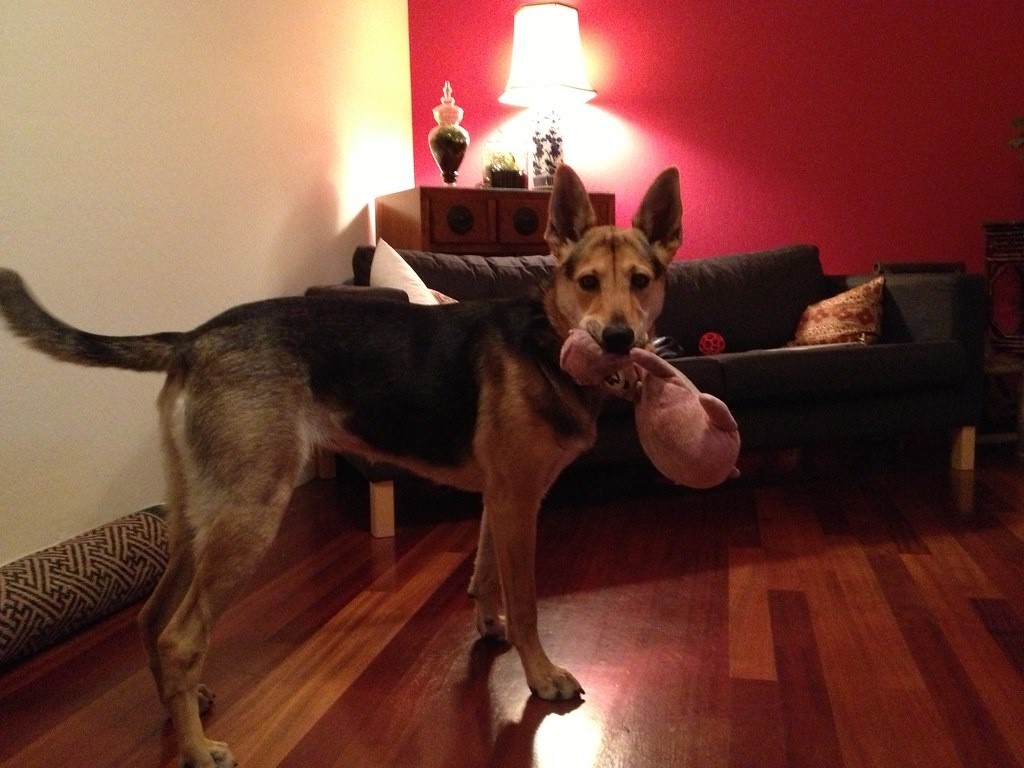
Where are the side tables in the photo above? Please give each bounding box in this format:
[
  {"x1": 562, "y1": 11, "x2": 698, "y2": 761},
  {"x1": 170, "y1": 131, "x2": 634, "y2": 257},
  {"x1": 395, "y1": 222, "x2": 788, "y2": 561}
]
[{"x1": 374, "y1": 185, "x2": 616, "y2": 256}]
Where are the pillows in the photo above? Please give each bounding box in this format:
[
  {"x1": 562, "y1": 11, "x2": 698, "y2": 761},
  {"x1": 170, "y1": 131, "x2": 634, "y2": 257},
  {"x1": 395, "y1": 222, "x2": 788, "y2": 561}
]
[
  {"x1": 369, "y1": 237, "x2": 459, "y2": 305},
  {"x1": 781, "y1": 276, "x2": 885, "y2": 347}
]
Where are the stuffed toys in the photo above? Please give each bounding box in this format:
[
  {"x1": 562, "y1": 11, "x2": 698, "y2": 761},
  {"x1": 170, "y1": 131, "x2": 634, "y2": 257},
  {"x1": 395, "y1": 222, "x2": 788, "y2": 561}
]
[{"x1": 558, "y1": 328, "x2": 741, "y2": 490}]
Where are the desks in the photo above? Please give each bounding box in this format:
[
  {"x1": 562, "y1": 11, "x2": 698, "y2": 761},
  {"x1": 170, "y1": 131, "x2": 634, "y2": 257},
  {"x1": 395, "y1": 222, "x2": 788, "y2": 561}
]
[{"x1": 984, "y1": 222, "x2": 1024, "y2": 409}]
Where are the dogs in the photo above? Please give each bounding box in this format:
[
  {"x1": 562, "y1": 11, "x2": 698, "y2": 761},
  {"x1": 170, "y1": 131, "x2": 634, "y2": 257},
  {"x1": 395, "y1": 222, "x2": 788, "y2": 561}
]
[{"x1": 2, "y1": 161, "x2": 681, "y2": 768}]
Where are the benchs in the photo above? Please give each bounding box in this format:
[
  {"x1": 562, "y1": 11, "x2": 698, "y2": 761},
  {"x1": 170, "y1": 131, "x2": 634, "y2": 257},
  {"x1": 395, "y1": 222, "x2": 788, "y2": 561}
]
[{"x1": 303, "y1": 242, "x2": 988, "y2": 539}]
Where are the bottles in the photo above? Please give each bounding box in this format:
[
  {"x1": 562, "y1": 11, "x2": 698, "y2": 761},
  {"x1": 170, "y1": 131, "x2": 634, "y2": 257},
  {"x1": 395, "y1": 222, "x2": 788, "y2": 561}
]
[{"x1": 427, "y1": 81, "x2": 471, "y2": 188}]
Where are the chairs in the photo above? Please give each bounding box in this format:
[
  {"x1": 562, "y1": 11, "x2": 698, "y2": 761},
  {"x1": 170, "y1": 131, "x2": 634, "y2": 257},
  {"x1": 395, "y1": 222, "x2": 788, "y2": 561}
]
[{"x1": 872, "y1": 259, "x2": 1024, "y2": 457}]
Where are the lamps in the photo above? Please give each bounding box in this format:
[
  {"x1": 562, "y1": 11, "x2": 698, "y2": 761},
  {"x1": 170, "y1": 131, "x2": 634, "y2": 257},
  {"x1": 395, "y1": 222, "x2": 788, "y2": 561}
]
[{"x1": 498, "y1": 1, "x2": 599, "y2": 190}]
